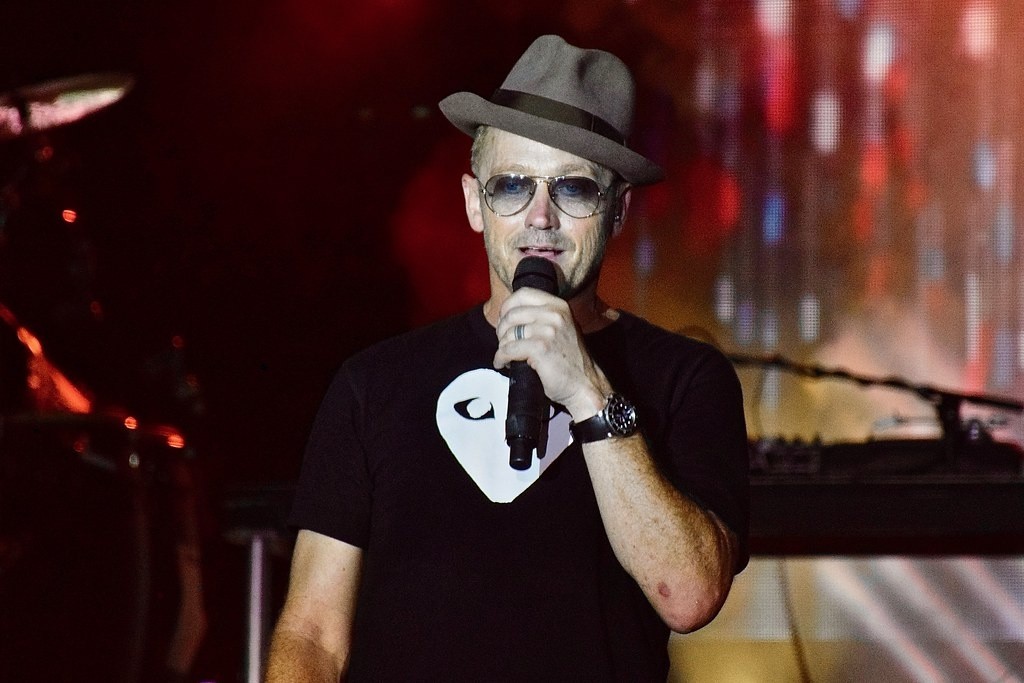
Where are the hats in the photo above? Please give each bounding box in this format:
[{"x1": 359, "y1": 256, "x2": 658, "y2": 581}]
[{"x1": 438, "y1": 35, "x2": 662, "y2": 186}]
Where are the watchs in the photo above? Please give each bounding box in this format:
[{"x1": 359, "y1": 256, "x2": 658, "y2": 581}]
[{"x1": 568, "y1": 391, "x2": 652, "y2": 444}]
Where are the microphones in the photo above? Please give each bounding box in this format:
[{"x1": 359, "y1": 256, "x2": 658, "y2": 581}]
[{"x1": 505, "y1": 255, "x2": 560, "y2": 471}]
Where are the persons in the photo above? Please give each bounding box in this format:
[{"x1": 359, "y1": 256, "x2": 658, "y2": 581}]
[{"x1": 261, "y1": 34, "x2": 751, "y2": 683}]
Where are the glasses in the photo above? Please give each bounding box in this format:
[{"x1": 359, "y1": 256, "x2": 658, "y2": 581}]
[{"x1": 477, "y1": 173, "x2": 614, "y2": 219}]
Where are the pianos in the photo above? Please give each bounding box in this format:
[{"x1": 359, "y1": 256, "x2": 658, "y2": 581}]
[{"x1": 735, "y1": 427, "x2": 1024, "y2": 561}]
